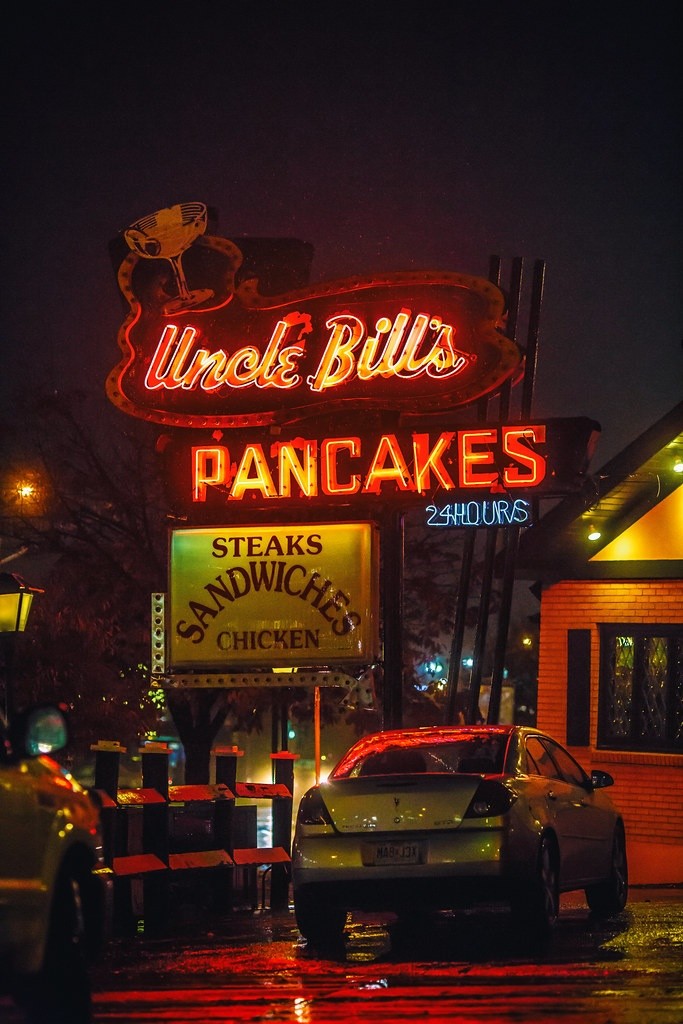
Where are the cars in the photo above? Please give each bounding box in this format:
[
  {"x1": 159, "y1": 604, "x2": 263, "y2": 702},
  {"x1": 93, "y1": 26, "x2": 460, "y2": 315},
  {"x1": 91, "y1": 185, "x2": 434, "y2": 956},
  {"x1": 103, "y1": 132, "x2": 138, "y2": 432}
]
[
  {"x1": 289, "y1": 723, "x2": 630, "y2": 941},
  {"x1": 0, "y1": 679, "x2": 121, "y2": 1024}
]
